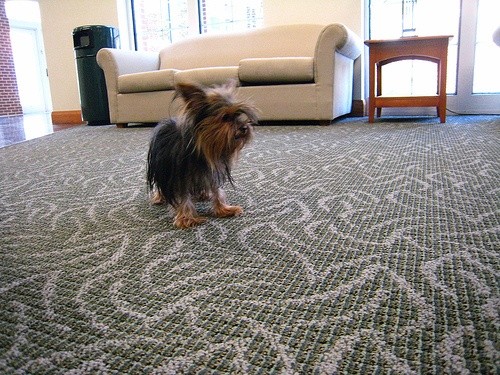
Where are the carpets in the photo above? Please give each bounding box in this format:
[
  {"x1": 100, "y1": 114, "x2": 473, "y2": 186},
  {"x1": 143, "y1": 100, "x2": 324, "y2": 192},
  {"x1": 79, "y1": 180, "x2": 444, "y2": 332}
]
[{"x1": 1, "y1": 116, "x2": 499, "y2": 375}]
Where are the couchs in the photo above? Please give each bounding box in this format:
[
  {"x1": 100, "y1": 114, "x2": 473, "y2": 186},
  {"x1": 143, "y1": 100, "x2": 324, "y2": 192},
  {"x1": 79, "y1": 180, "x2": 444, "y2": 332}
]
[{"x1": 96, "y1": 20, "x2": 362, "y2": 129}]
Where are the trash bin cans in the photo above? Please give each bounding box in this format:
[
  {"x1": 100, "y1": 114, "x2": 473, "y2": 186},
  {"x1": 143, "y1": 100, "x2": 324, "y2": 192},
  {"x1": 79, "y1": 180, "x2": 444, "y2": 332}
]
[{"x1": 72, "y1": 24, "x2": 120, "y2": 125}]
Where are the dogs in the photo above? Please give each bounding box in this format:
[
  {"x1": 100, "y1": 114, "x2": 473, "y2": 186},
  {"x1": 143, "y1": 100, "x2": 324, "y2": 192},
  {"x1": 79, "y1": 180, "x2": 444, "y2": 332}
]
[{"x1": 145, "y1": 77, "x2": 264, "y2": 231}]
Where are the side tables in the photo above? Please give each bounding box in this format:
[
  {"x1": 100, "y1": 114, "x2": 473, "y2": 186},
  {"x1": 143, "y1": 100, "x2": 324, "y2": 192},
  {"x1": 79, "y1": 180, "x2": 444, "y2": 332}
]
[{"x1": 364, "y1": 33, "x2": 455, "y2": 125}]
